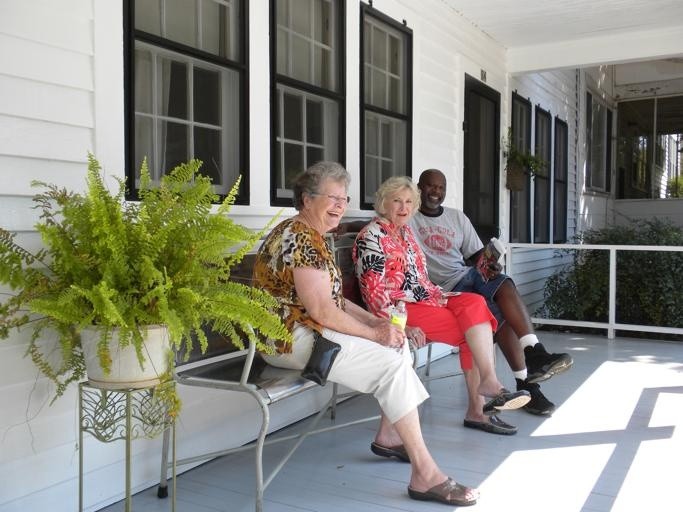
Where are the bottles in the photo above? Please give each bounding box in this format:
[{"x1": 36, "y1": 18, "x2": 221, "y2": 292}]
[{"x1": 389, "y1": 302, "x2": 407, "y2": 331}]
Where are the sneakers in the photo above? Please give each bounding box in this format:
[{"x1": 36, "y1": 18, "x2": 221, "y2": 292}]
[
  {"x1": 482, "y1": 389, "x2": 531, "y2": 415},
  {"x1": 464, "y1": 413, "x2": 518, "y2": 434}
]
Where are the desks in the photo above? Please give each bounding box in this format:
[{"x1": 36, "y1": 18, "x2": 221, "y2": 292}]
[{"x1": 77, "y1": 384, "x2": 178, "y2": 512}]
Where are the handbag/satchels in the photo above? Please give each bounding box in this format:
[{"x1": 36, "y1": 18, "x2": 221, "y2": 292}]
[{"x1": 301, "y1": 330, "x2": 341, "y2": 387}]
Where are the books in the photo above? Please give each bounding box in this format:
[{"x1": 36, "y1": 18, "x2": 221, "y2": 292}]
[{"x1": 475, "y1": 237, "x2": 506, "y2": 285}]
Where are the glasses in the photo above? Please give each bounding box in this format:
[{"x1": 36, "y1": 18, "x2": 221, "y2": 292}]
[{"x1": 310, "y1": 191, "x2": 348, "y2": 205}]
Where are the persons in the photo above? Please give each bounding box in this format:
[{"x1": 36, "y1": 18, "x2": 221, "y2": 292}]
[
  {"x1": 247, "y1": 159, "x2": 480, "y2": 505},
  {"x1": 353, "y1": 174, "x2": 531, "y2": 436},
  {"x1": 407, "y1": 169, "x2": 575, "y2": 416}
]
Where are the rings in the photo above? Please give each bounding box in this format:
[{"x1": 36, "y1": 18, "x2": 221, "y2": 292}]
[
  {"x1": 492, "y1": 273, "x2": 494, "y2": 277},
  {"x1": 414, "y1": 333, "x2": 417, "y2": 337}
]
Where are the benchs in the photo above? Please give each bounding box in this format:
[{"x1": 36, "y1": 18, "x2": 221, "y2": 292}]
[{"x1": 158, "y1": 241, "x2": 495, "y2": 511}]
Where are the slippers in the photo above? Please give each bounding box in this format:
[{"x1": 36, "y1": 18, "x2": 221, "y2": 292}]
[
  {"x1": 371, "y1": 442, "x2": 412, "y2": 463},
  {"x1": 407, "y1": 477, "x2": 480, "y2": 505}
]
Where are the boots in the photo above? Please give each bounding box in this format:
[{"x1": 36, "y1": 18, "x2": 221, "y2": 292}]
[
  {"x1": 514, "y1": 376, "x2": 555, "y2": 416},
  {"x1": 523, "y1": 343, "x2": 572, "y2": 384}
]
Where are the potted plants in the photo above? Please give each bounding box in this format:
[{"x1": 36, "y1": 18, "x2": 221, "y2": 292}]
[{"x1": 1, "y1": 149, "x2": 297, "y2": 455}]
